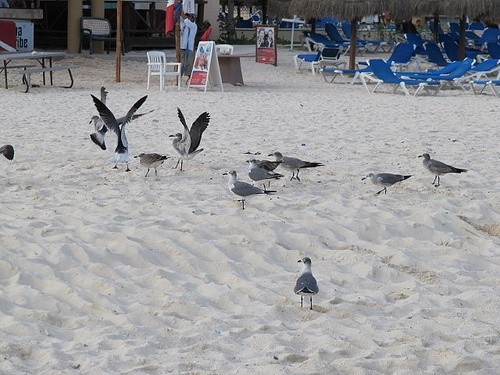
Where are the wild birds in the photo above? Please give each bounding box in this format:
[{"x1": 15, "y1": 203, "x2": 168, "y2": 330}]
[
  {"x1": 361, "y1": 173, "x2": 413, "y2": 194},
  {"x1": 88, "y1": 86, "x2": 207, "y2": 176},
  {"x1": 221, "y1": 152, "x2": 326, "y2": 209},
  {"x1": 418, "y1": 153, "x2": 470, "y2": 189},
  {"x1": 293, "y1": 256, "x2": 320, "y2": 311},
  {"x1": 0, "y1": 144, "x2": 14, "y2": 162}
]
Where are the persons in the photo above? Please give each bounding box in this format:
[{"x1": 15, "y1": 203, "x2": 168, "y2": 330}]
[
  {"x1": 180, "y1": 10, "x2": 198, "y2": 76},
  {"x1": 257, "y1": 29, "x2": 273, "y2": 47},
  {"x1": 415, "y1": 20, "x2": 422, "y2": 28}
]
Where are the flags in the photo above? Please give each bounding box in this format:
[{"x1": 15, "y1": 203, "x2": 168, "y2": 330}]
[{"x1": 165, "y1": 0, "x2": 174, "y2": 35}]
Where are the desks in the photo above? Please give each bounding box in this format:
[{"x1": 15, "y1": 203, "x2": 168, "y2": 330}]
[
  {"x1": 218, "y1": 53, "x2": 244, "y2": 86},
  {"x1": 0, "y1": 51, "x2": 66, "y2": 92}
]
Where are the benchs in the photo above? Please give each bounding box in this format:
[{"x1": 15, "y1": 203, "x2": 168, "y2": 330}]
[
  {"x1": 78, "y1": 17, "x2": 125, "y2": 56},
  {"x1": 1, "y1": 62, "x2": 81, "y2": 93}
]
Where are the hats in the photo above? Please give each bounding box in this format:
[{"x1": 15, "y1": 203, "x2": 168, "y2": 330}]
[{"x1": 186, "y1": 13, "x2": 196, "y2": 18}]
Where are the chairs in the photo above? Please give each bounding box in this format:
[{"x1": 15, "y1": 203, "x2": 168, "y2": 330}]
[
  {"x1": 147, "y1": 50, "x2": 183, "y2": 92},
  {"x1": 237, "y1": 18, "x2": 500, "y2": 96}
]
